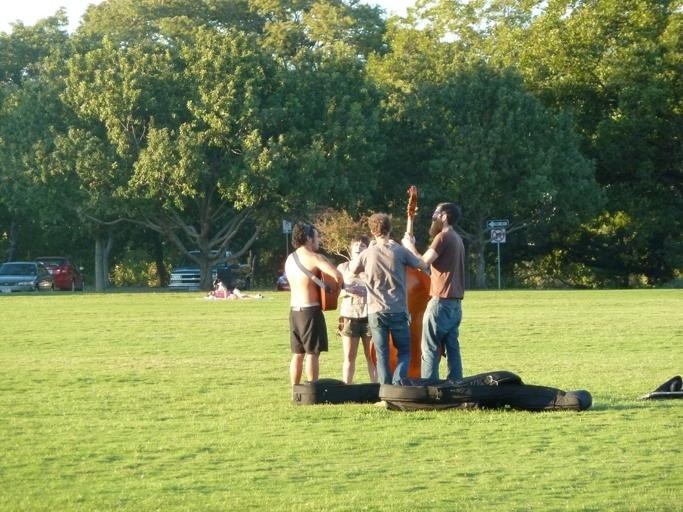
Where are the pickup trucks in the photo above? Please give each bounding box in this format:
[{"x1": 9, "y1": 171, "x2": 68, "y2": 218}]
[{"x1": 169, "y1": 248, "x2": 253, "y2": 294}]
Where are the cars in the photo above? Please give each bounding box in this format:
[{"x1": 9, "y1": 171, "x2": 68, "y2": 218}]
[
  {"x1": 277, "y1": 274, "x2": 293, "y2": 292},
  {"x1": 0, "y1": 261, "x2": 57, "y2": 294},
  {"x1": 34, "y1": 256, "x2": 87, "y2": 293}
]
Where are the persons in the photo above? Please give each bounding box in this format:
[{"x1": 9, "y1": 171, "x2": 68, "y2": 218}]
[
  {"x1": 398, "y1": 201, "x2": 467, "y2": 382},
  {"x1": 282, "y1": 221, "x2": 345, "y2": 401},
  {"x1": 206, "y1": 287, "x2": 263, "y2": 300},
  {"x1": 333, "y1": 236, "x2": 378, "y2": 384},
  {"x1": 349, "y1": 213, "x2": 425, "y2": 384}
]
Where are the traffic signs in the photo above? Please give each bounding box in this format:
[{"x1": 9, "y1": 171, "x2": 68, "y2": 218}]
[{"x1": 487, "y1": 219, "x2": 510, "y2": 230}]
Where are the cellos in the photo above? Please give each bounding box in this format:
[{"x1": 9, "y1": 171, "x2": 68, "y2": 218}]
[{"x1": 369, "y1": 186, "x2": 442, "y2": 378}]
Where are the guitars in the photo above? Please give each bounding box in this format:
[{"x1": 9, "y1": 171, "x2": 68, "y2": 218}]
[{"x1": 319, "y1": 271, "x2": 337, "y2": 310}]
